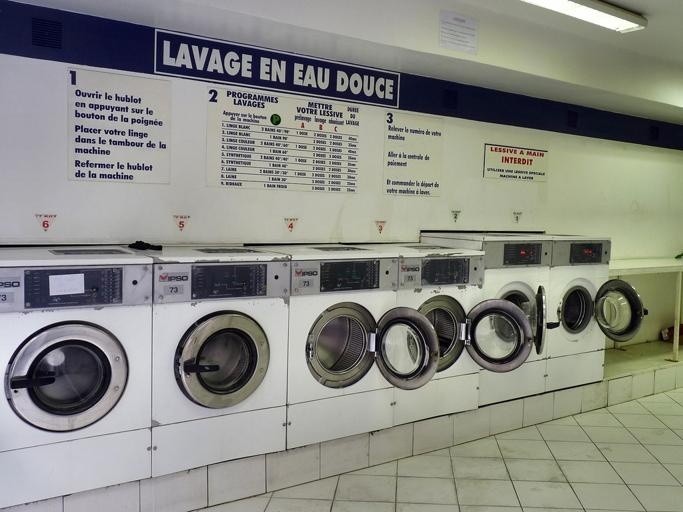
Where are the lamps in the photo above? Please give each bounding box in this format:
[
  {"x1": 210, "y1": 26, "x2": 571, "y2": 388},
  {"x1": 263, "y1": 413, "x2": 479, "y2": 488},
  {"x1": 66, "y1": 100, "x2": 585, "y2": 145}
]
[{"x1": 520, "y1": 1, "x2": 648, "y2": 36}]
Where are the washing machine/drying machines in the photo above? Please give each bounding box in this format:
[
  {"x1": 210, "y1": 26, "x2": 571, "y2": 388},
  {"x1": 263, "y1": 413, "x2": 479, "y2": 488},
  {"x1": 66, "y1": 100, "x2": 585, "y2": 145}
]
[
  {"x1": 242, "y1": 245, "x2": 441, "y2": 451},
  {"x1": 527, "y1": 233, "x2": 648, "y2": 395},
  {"x1": 1, "y1": 246, "x2": 154, "y2": 510},
  {"x1": 127, "y1": 245, "x2": 291, "y2": 480},
  {"x1": 350, "y1": 243, "x2": 534, "y2": 426},
  {"x1": 421, "y1": 232, "x2": 562, "y2": 406}
]
